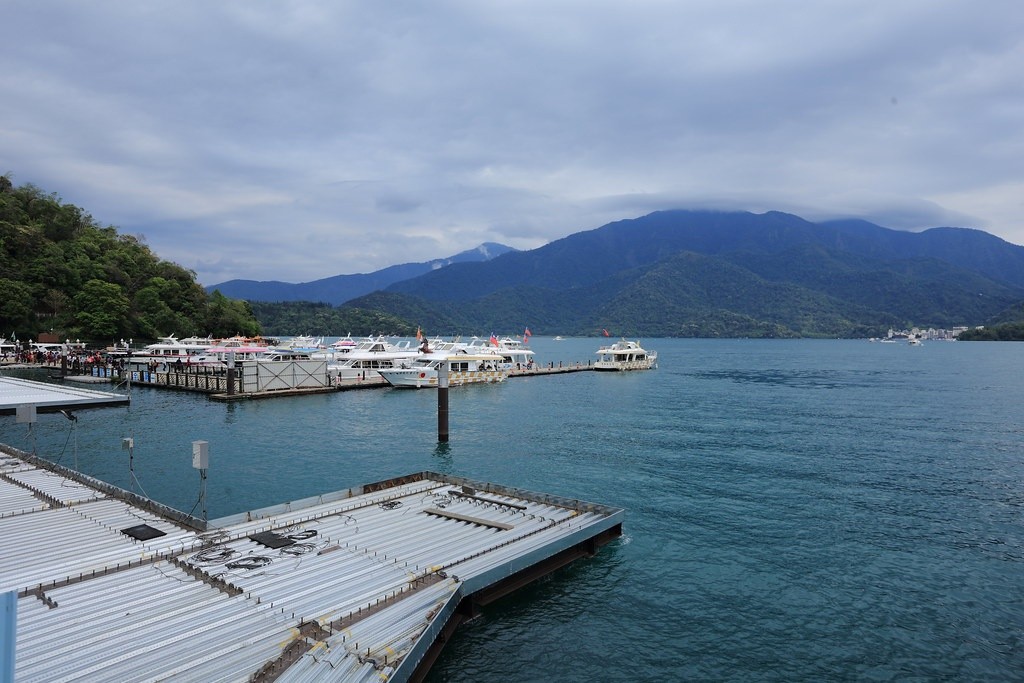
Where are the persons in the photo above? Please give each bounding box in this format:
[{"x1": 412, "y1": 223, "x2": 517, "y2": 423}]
[
  {"x1": 479, "y1": 364, "x2": 497, "y2": 371},
  {"x1": 187, "y1": 349, "x2": 195, "y2": 355},
  {"x1": 149, "y1": 358, "x2": 158, "y2": 374},
  {"x1": 163, "y1": 357, "x2": 167, "y2": 372},
  {"x1": 527, "y1": 357, "x2": 533, "y2": 369},
  {"x1": 186, "y1": 357, "x2": 191, "y2": 370},
  {"x1": 176, "y1": 357, "x2": 181, "y2": 371},
  {"x1": 15, "y1": 350, "x2": 126, "y2": 368},
  {"x1": 2, "y1": 351, "x2": 8, "y2": 362}
]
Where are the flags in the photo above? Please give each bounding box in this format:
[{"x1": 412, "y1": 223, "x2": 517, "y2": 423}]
[
  {"x1": 11, "y1": 332, "x2": 15, "y2": 341},
  {"x1": 524, "y1": 327, "x2": 531, "y2": 343},
  {"x1": 602, "y1": 329, "x2": 609, "y2": 337},
  {"x1": 490, "y1": 332, "x2": 499, "y2": 347}
]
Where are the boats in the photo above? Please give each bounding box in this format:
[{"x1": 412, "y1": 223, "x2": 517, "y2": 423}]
[
  {"x1": 144, "y1": 333, "x2": 534, "y2": 376},
  {"x1": 593, "y1": 336, "x2": 658, "y2": 371},
  {"x1": 376, "y1": 352, "x2": 510, "y2": 390},
  {"x1": 326, "y1": 341, "x2": 425, "y2": 386},
  {"x1": 879, "y1": 337, "x2": 896, "y2": 344},
  {"x1": 907, "y1": 340, "x2": 924, "y2": 347},
  {"x1": 869, "y1": 338, "x2": 875, "y2": 342}
]
